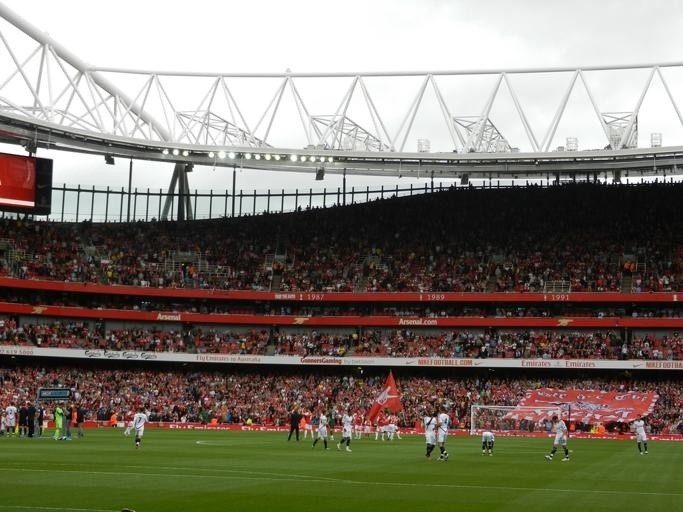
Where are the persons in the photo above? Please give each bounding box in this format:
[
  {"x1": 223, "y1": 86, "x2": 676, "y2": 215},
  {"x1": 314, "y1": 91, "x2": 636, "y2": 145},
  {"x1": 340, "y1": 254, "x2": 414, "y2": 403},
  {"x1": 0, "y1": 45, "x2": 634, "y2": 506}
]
[
  {"x1": 431, "y1": 404, "x2": 449, "y2": 463},
  {"x1": 630, "y1": 414, "x2": 649, "y2": 455},
  {"x1": 0, "y1": 293, "x2": 683, "y2": 441},
  {"x1": 335, "y1": 409, "x2": 352, "y2": 453},
  {"x1": 541, "y1": 414, "x2": 569, "y2": 461},
  {"x1": 421, "y1": 408, "x2": 436, "y2": 460},
  {"x1": 310, "y1": 409, "x2": 331, "y2": 450},
  {"x1": 132, "y1": 405, "x2": 148, "y2": 449},
  {"x1": 480, "y1": 430, "x2": 494, "y2": 456},
  {"x1": 1, "y1": 179, "x2": 682, "y2": 293}
]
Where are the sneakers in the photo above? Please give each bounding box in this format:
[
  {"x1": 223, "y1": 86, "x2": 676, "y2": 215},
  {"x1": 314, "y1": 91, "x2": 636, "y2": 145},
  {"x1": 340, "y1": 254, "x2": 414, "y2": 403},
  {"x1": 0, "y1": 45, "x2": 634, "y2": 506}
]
[
  {"x1": 424, "y1": 450, "x2": 448, "y2": 462},
  {"x1": 336, "y1": 444, "x2": 341, "y2": 451},
  {"x1": 0, "y1": 430, "x2": 83, "y2": 441},
  {"x1": 560, "y1": 458, "x2": 568, "y2": 462},
  {"x1": 354, "y1": 436, "x2": 402, "y2": 441},
  {"x1": 544, "y1": 456, "x2": 552, "y2": 461},
  {"x1": 346, "y1": 447, "x2": 352, "y2": 452}
]
